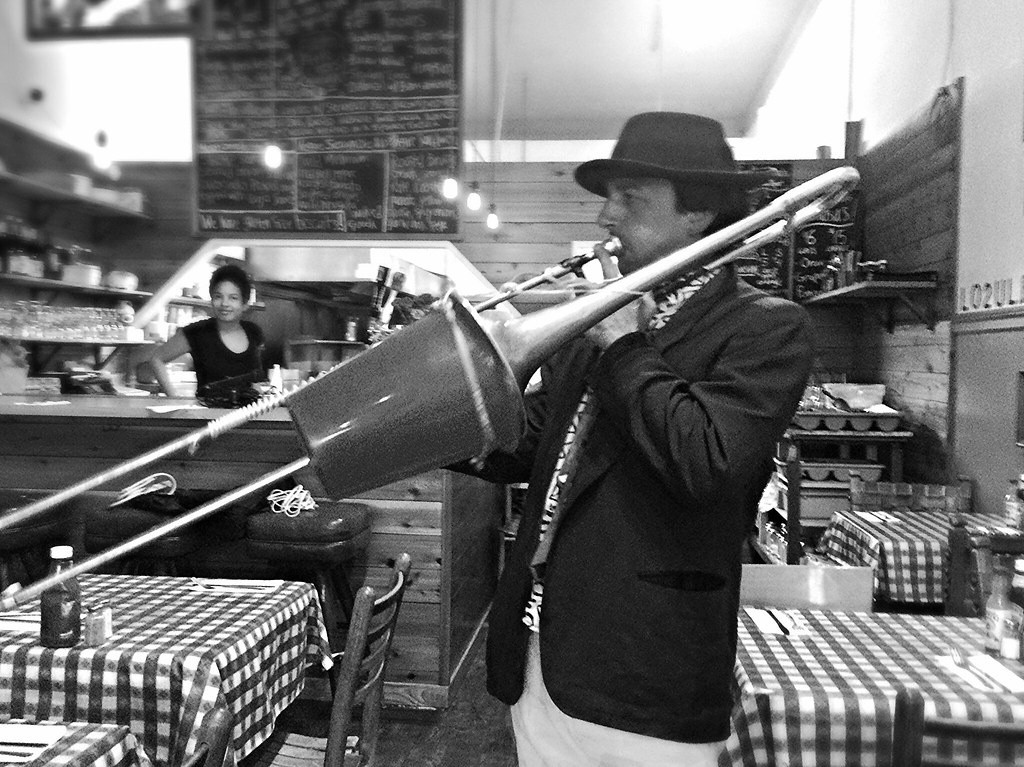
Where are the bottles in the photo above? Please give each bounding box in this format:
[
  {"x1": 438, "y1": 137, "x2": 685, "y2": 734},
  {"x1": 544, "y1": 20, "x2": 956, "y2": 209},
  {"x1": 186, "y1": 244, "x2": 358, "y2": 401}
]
[
  {"x1": 41, "y1": 546, "x2": 81, "y2": 649},
  {"x1": 986, "y1": 582, "x2": 1011, "y2": 652},
  {"x1": 1003, "y1": 480, "x2": 1024, "y2": 529},
  {"x1": 0, "y1": 216, "x2": 44, "y2": 278}
]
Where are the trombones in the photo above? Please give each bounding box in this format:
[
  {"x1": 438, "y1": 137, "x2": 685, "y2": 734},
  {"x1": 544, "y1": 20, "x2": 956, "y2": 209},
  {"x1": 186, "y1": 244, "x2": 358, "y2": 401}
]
[{"x1": 1, "y1": 164, "x2": 861, "y2": 618}]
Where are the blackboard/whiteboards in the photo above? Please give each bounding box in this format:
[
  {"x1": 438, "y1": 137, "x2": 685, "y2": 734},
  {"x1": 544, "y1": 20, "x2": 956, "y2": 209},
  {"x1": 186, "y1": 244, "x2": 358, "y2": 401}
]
[
  {"x1": 189, "y1": 2, "x2": 467, "y2": 241},
  {"x1": 737, "y1": 164, "x2": 795, "y2": 301},
  {"x1": 797, "y1": 195, "x2": 866, "y2": 299}
]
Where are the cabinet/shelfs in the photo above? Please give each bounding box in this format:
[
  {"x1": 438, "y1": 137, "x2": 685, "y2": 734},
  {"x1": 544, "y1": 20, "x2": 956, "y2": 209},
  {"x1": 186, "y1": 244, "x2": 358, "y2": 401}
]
[
  {"x1": 0, "y1": 168, "x2": 155, "y2": 297},
  {"x1": 747, "y1": 430, "x2": 920, "y2": 564}
]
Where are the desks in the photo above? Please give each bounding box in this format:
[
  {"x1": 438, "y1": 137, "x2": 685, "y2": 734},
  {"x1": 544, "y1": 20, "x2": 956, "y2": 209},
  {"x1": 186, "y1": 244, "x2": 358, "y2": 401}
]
[
  {"x1": 720, "y1": 606, "x2": 1024, "y2": 767},
  {"x1": 0, "y1": 335, "x2": 156, "y2": 403},
  {"x1": 817, "y1": 511, "x2": 1024, "y2": 605},
  {"x1": 0, "y1": 571, "x2": 334, "y2": 767}
]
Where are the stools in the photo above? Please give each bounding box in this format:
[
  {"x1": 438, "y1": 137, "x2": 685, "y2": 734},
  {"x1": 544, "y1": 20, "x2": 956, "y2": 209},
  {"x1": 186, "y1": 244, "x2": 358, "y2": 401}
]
[
  {"x1": 246, "y1": 502, "x2": 371, "y2": 703},
  {"x1": 81, "y1": 511, "x2": 165, "y2": 576},
  {"x1": 0, "y1": 490, "x2": 66, "y2": 590}
]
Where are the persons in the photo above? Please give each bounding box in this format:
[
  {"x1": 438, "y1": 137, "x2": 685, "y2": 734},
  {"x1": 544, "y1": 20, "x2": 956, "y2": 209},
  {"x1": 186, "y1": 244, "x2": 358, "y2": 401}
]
[
  {"x1": 442, "y1": 112, "x2": 817, "y2": 767},
  {"x1": 149, "y1": 264, "x2": 266, "y2": 412}
]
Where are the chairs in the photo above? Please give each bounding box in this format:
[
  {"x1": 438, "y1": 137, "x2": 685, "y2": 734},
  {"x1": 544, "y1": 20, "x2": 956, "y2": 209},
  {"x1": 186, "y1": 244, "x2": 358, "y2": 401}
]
[
  {"x1": 894, "y1": 691, "x2": 1024, "y2": 767},
  {"x1": 848, "y1": 470, "x2": 972, "y2": 512},
  {"x1": 740, "y1": 564, "x2": 876, "y2": 615},
  {"x1": 237, "y1": 554, "x2": 412, "y2": 766},
  {"x1": 944, "y1": 518, "x2": 1024, "y2": 615}
]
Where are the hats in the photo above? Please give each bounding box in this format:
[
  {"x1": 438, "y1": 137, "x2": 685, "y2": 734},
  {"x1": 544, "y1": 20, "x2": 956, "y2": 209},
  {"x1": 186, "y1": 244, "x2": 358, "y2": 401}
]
[{"x1": 574, "y1": 111, "x2": 778, "y2": 199}]
